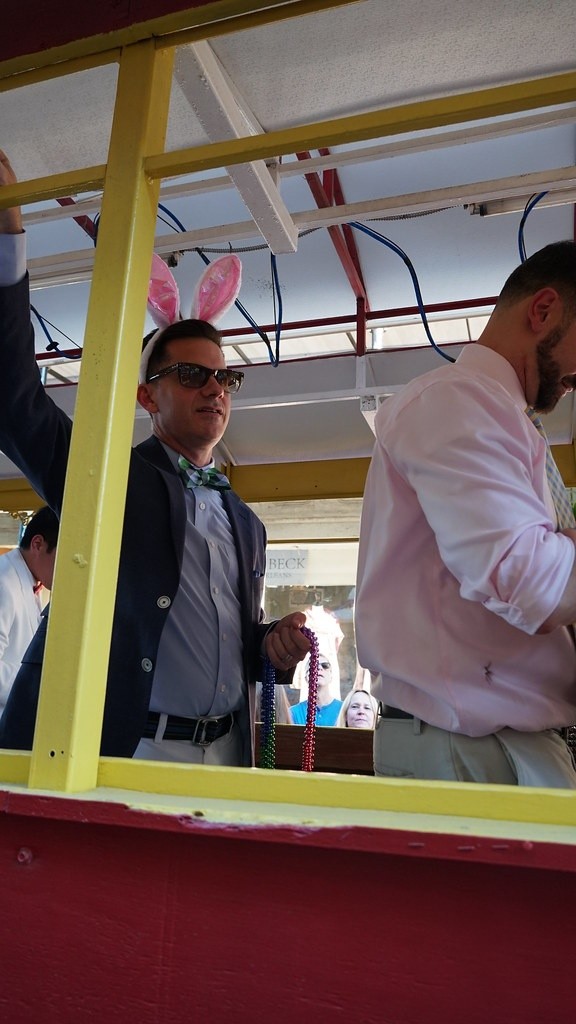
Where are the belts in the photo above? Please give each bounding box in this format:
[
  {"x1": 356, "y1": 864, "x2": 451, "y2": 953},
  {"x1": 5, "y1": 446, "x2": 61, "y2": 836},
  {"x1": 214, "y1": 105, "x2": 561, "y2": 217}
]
[
  {"x1": 141, "y1": 711, "x2": 239, "y2": 745},
  {"x1": 379, "y1": 701, "x2": 414, "y2": 718}
]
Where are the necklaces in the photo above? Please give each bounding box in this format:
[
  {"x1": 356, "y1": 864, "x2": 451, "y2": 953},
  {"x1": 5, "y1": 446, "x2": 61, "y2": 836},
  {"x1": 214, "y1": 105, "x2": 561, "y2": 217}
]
[{"x1": 258, "y1": 625, "x2": 320, "y2": 773}]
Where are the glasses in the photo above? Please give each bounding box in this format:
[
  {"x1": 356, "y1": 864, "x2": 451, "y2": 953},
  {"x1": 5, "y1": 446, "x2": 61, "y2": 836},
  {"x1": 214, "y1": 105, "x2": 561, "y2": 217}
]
[
  {"x1": 306, "y1": 662, "x2": 331, "y2": 672},
  {"x1": 147, "y1": 363, "x2": 245, "y2": 394}
]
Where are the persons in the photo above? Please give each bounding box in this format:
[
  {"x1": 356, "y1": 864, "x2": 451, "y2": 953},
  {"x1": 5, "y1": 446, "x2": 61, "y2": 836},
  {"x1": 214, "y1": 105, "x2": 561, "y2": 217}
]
[
  {"x1": 353, "y1": 237, "x2": 576, "y2": 794},
  {"x1": 0, "y1": 505, "x2": 60, "y2": 716},
  {"x1": 1, "y1": 149, "x2": 315, "y2": 769},
  {"x1": 288, "y1": 653, "x2": 349, "y2": 729},
  {"x1": 333, "y1": 687, "x2": 381, "y2": 731}
]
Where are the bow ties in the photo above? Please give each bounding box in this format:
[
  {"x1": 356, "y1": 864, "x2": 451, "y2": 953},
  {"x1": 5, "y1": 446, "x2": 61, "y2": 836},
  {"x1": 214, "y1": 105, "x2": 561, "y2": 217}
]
[{"x1": 178, "y1": 454, "x2": 231, "y2": 490}]
